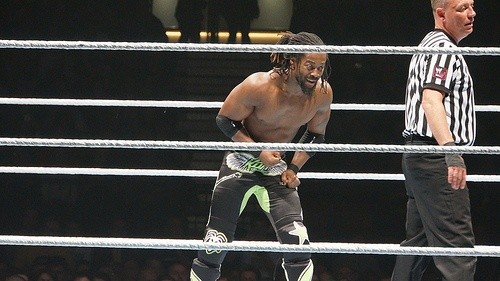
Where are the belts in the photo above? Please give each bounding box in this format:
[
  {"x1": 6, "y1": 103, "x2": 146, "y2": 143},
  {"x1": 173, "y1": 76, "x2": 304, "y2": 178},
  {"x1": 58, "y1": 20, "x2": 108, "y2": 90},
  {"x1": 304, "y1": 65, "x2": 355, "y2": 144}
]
[{"x1": 404, "y1": 136, "x2": 436, "y2": 143}]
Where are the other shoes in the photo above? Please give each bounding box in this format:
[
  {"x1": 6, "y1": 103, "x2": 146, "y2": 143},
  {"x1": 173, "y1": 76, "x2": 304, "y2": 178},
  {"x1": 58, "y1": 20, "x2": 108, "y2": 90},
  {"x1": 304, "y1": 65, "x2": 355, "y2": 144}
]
[
  {"x1": 179, "y1": 34, "x2": 200, "y2": 43},
  {"x1": 241, "y1": 34, "x2": 251, "y2": 44},
  {"x1": 226, "y1": 34, "x2": 236, "y2": 44}
]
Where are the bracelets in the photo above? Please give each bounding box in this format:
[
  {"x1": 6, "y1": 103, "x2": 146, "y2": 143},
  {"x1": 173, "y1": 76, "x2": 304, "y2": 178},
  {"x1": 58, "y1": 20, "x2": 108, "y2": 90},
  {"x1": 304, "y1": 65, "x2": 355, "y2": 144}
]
[
  {"x1": 250, "y1": 150, "x2": 262, "y2": 158},
  {"x1": 288, "y1": 164, "x2": 299, "y2": 174}
]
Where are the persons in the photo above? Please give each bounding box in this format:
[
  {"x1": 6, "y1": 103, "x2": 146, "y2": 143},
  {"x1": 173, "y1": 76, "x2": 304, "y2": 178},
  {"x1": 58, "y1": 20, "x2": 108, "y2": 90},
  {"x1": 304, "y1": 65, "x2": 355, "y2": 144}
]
[
  {"x1": 190, "y1": 31, "x2": 334, "y2": 281},
  {"x1": 0, "y1": 212, "x2": 391, "y2": 281},
  {"x1": 174, "y1": 0, "x2": 260, "y2": 43},
  {"x1": 390, "y1": 0, "x2": 477, "y2": 281}
]
